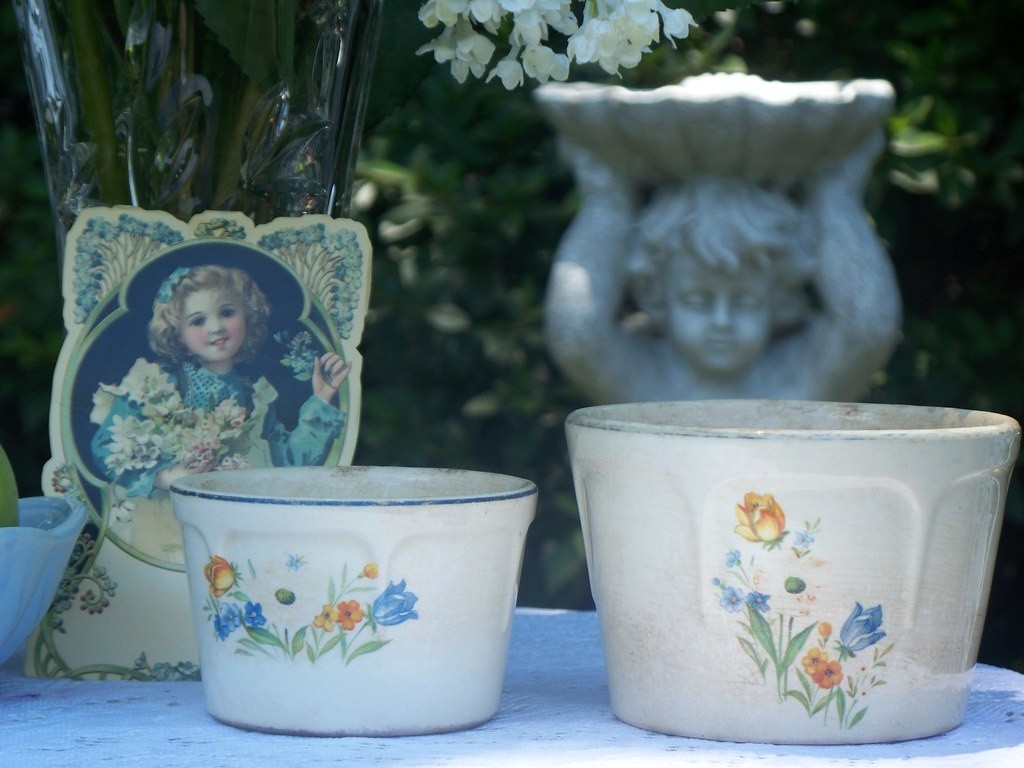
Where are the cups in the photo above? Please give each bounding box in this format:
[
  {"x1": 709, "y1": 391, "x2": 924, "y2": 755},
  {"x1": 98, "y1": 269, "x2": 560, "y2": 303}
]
[{"x1": 9, "y1": 0, "x2": 390, "y2": 258}]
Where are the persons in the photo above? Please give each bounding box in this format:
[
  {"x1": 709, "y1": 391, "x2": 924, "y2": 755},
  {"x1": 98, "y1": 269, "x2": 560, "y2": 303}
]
[{"x1": 544, "y1": 133, "x2": 900, "y2": 402}]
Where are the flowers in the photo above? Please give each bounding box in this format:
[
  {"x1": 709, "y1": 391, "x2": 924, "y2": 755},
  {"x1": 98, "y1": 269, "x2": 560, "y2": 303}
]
[{"x1": 415, "y1": 0, "x2": 698, "y2": 91}]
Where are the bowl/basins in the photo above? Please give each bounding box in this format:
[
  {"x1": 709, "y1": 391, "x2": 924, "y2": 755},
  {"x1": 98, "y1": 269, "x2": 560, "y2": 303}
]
[
  {"x1": 0, "y1": 495, "x2": 90, "y2": 663},
  {"x1": 559, "y1": 397, "x2": 1022, "y2": 750},
  {"x1": 164, "y1": 462, "x2": 544, "y2": 740}
]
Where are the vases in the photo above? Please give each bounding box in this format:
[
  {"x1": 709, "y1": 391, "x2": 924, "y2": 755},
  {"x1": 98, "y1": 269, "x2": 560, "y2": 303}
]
[{"x1": 12, "y1": 0, "x2": 382, "y2": 272}]
